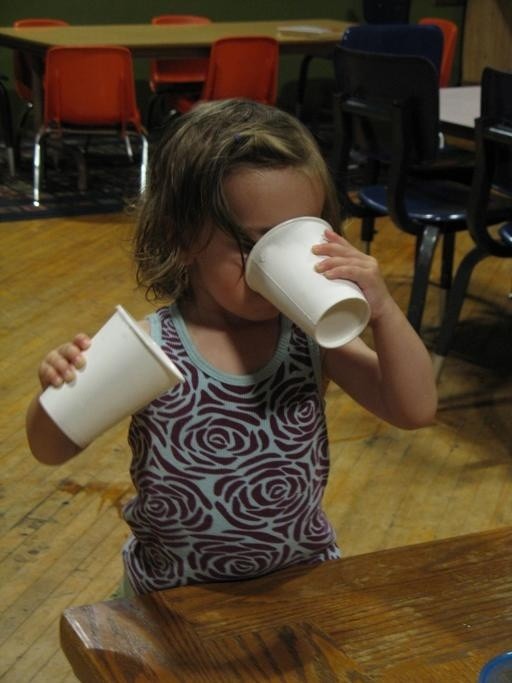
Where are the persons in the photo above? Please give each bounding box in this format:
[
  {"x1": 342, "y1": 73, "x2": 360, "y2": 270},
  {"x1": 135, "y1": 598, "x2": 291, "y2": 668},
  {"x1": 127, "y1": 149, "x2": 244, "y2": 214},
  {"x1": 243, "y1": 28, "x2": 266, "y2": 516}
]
[{"x1": 23, "y1": 93, "x2": 442, "y2": 599}]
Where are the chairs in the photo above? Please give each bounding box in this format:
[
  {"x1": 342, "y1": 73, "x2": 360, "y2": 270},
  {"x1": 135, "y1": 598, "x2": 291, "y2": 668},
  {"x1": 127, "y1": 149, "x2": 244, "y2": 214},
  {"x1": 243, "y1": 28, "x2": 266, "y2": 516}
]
[
  {"x1": 146, "y1": 15, "x2": 215, "y2": 123},
  {"x1": 435, "y1": 66, "x2": 509, "y2": 354},
  {"x1": 28, "y1": 45, "x2": 154, "y2": 211},
  {"x1": 166, "y1": 35, "x2": 281, "y2": 123},
  {"x1": 296, "y1": 18, "x2": 459, "y2": 118},
  {"x1": 10, "y1": 19, "x2": 93, "y2": 195},
  {"x1": 327, "y1": 23, "x2": 479, "y2": 334}
]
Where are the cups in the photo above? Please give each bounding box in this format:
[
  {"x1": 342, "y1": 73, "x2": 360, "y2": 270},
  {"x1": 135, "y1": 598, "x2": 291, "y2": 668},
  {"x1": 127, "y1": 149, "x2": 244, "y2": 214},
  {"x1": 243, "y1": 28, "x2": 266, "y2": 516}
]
[
  {"x1": 38, "y1": 303, "x2": 188, "y2": 449},
  {"x1": 243, "y1": 217, "x2": 373, "y2": 350}
]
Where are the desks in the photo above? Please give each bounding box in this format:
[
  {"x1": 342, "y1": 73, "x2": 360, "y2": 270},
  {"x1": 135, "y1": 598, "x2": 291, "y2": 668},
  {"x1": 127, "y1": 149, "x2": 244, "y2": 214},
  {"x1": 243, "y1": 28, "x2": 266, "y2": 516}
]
[
  {"x1": 2, "y1": 13, "x2": 349, "y2": 192},
  {"x1": 432, "y1": 82, "x2": 511, "y2": 291},
  {"x1": 57, "y1": 526, "x2": 511, "y2": 682}
]
